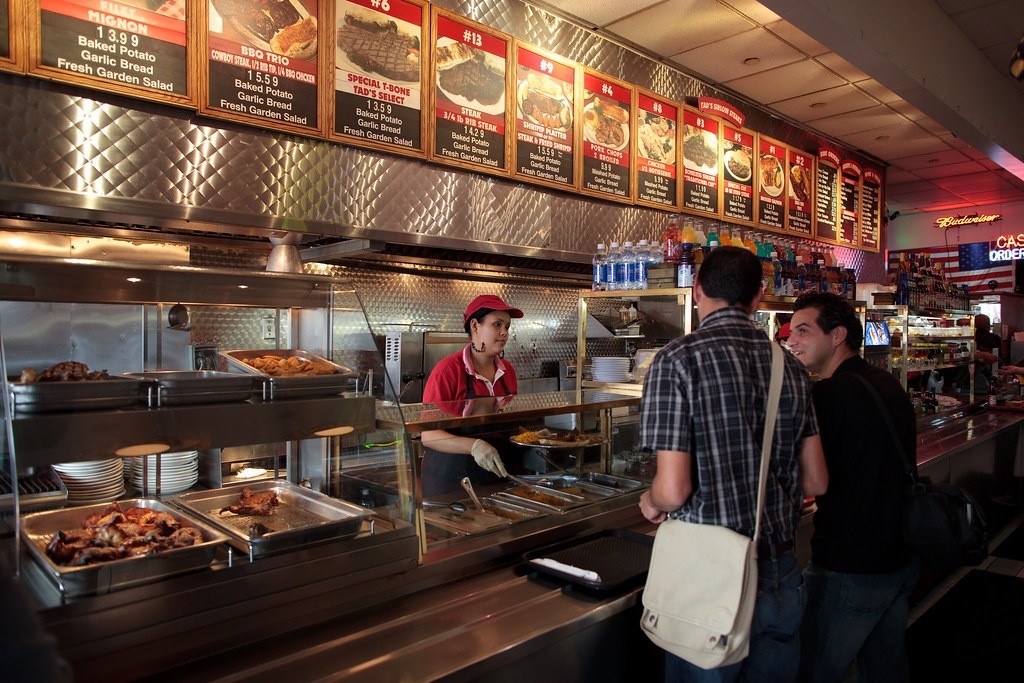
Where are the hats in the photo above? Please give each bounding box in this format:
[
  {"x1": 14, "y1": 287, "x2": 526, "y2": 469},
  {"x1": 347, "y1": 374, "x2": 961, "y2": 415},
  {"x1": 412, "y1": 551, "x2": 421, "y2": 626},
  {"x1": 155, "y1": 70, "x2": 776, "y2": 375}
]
[
  {"x1": 464, "y1": 295, "x2": 524, "y2": 326},
  {"x1": 775, "y1": 323, "x2": 791, "y2": 339}
]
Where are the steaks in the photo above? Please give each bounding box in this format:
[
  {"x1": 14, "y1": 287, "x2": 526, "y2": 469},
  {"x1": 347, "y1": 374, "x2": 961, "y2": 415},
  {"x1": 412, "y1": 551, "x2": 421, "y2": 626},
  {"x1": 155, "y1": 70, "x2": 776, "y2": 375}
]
[
  {"x1": 683, "y1": 140, "x2": 717, "y2": 168},
  {"x1": 527, "y1": 91, "x2": 560, "y2": 115},
  {"x1": 728, "y1": 160, "x2": 750, "y2": 178},
  {"x1": 336, "y1": 25, "x2": 420, "y2": 82},
  {"x1": 440, "y1": 61, "x2": 505, "y2": 105}
]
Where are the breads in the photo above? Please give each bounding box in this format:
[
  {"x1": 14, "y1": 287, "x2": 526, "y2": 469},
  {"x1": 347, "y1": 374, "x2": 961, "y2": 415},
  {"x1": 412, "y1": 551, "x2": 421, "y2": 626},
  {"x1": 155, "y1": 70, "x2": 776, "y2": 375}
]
[
  {"x1": 519, "y1": 426, "x2": 557, "y2": 438},
  {"x1": 539, "y1": 438, "x2": 589, "y2": 445},
  {"x1": 592, "y1": 98, "x2": 629, "y2": 124},
  {"x1": 271, "y1": 15, "x2": 318, "y2": 60}
]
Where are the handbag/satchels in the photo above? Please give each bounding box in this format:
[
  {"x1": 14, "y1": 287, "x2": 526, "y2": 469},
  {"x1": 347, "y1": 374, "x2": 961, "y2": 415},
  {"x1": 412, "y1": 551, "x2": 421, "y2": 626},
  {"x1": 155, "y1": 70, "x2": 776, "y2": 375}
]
[{"x1": 640, "y1": 519, "x2": 758, "y2": 670}]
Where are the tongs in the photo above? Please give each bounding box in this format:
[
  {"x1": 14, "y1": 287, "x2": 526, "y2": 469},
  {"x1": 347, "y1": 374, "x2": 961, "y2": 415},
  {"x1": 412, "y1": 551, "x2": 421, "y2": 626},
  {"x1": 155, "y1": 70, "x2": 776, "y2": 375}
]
[
  {"x1": 422, "y1": 500, "x2": 466, "y2": 512},
  {"x1": 517, "y1": 472, "x2": 580, "y2": 489}
]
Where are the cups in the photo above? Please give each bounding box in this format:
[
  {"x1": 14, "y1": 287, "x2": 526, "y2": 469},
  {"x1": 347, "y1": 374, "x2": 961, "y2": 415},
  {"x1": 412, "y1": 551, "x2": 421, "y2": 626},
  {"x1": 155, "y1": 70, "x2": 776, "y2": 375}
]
[
  {"x1": 628, "y1": 325, "x2": 639, "y2": 336},
  {"x1": 1014, "y1": 332, "x2": 1024, "y2": 341}
]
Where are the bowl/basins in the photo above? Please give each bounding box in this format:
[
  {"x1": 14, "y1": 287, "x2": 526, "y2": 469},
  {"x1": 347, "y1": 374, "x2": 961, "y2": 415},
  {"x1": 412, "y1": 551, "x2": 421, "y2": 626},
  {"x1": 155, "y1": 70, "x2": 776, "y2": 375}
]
[{"x1": 615, "y1": 329, "x2": 628, "y2": 335}]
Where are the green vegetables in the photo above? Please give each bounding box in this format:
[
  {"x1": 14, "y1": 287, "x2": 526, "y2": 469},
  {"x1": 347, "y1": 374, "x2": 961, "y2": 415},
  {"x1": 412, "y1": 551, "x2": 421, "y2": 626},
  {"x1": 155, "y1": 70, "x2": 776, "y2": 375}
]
[
  {"x1": 592, "y1": 106, "x2": 604, "y2": 116},
  {"x1": 644, "y1": 113, "x2": 672, "y2": 161},
  {"x1": 346, "y1": 15, "x2": 420, "y2": 72}
]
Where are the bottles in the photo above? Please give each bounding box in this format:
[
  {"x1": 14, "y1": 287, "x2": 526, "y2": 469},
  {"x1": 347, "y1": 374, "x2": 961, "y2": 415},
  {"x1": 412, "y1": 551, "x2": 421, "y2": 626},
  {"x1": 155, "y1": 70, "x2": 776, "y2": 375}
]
[
  {"x1": 988, "y1": 374, "x2": 1023, "y2": 405},
  {"x1": 592, "y1": 240, "x2": 663, "y2": 289},
  {"x1": 620, "y1": 303, "x2": 637, "y2": 329},
  {"x1": 663, "y1": 214, "x2": 856, "y2": 301},
  {"x1": 910, "y1": 387, "x2": 941, "y2": 418},
  {"x1": 896, "y1": 250, "x2": 970, "y2": 311}
]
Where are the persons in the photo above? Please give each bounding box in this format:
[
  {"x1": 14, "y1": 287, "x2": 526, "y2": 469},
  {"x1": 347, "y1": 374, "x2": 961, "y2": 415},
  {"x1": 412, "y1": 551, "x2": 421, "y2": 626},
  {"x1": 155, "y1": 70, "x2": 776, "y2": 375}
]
[
  {"x1": 786, "y1": 291, "x2": 920, "y2": 683},
  {"x1": 908, "y1": 309, "x2": 1024, "y2": 391},
  {"x1": 636, "y1": 242, "x2": 827, "y2": 683},
  {"x1": 420, "y1": 296, "x2": 526, "y2": 499}
]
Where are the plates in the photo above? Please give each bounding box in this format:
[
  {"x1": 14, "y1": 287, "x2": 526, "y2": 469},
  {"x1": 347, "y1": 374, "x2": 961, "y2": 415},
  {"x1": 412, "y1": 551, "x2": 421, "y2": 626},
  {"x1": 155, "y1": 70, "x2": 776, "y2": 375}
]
[
  {"x1": 724, "y1": 150, "x2": 751, "y2": 181},
  {"x1": 583, "y1": 102, "x2": 630, "y2": 151},
  {"x1": 211, "y1": 0, "x2": 422, "y2": 84},
  {"x1": 639, "y1": 123, "x2": 676, "y2": 164},
  {"x1": 53, "y1": 458, "x2": 127, "y2": 508},
  {"x1": 591, "y1": 391, "x2": 630, "y2": 403},
  {"x1": 683, "y1": 125, "x2": 718, "y2": 175},
  {"x1": 123, "y1": 450, "x2": 199, "y2": 496},
  {"x1": 760, "y1": 155, "x2": 785, "y2": 197},
  {"x1": 790, "y1": 165, "x2": 806, "y2": 199},
  {"x1": 591, "y1": 357, "x2": 634, "y2": 383},
  {"x1": 436, "y1": 36, "x2": 505, "y2": 115},
  {"x1": 517, "y1": 78, "x2": 573, "y2": 132}
]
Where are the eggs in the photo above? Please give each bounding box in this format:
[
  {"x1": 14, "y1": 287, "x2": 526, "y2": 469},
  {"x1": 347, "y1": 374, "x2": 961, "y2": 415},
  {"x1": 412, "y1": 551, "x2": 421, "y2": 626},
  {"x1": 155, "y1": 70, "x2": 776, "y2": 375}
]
[{"x1": 584, "y1": 108, "x2": 598, "y2": 127}]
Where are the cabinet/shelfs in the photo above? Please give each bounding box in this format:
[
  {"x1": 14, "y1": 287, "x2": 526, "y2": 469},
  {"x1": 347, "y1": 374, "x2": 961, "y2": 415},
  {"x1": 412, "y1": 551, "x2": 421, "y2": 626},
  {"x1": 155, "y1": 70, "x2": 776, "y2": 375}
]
[{"x1": 868, "y1": 304, "x2": 975, "y2": 404}]
